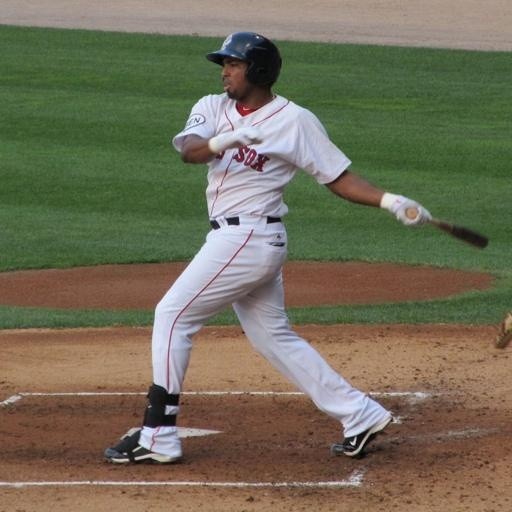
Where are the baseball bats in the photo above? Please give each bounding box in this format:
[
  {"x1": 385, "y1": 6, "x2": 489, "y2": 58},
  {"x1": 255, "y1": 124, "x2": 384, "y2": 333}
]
[{"x1": 405, "y1": 207, "x2": 489, "y2": 251}]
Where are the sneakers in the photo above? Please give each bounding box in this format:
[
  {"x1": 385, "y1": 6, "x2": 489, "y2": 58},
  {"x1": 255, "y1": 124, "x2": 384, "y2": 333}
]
[
  {"x1": 103, "y1": 427, "x2": 176, "y2": 464},
  {"x1": 342, "y1": 413, "x2": 393, "y2": 456}
]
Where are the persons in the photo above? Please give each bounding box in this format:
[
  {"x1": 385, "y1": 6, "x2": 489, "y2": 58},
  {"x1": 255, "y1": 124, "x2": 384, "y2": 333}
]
[{"x1": 102, "y1": 31, "x2": 434, "y2": 464}]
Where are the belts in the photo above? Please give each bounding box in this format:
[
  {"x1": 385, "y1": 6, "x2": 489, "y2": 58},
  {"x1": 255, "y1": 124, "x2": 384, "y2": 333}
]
[{"x1": 209, "y1": 215, "x2": 281, "y2": 230}]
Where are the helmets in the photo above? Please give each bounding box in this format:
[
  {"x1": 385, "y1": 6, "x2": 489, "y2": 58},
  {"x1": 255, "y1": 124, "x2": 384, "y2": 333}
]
[{"x1": 206, "y1": 31, "x2": 281, "y2": 87}]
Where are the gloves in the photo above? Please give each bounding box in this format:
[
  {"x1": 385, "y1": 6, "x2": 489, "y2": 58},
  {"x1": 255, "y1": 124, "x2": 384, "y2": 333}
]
[
  {"x1": 379, "y1": 191, "x2": 433, "y2": 226},
  {"x1": 208, "y1": 127, "x2": 264, "y2": 154}
]
